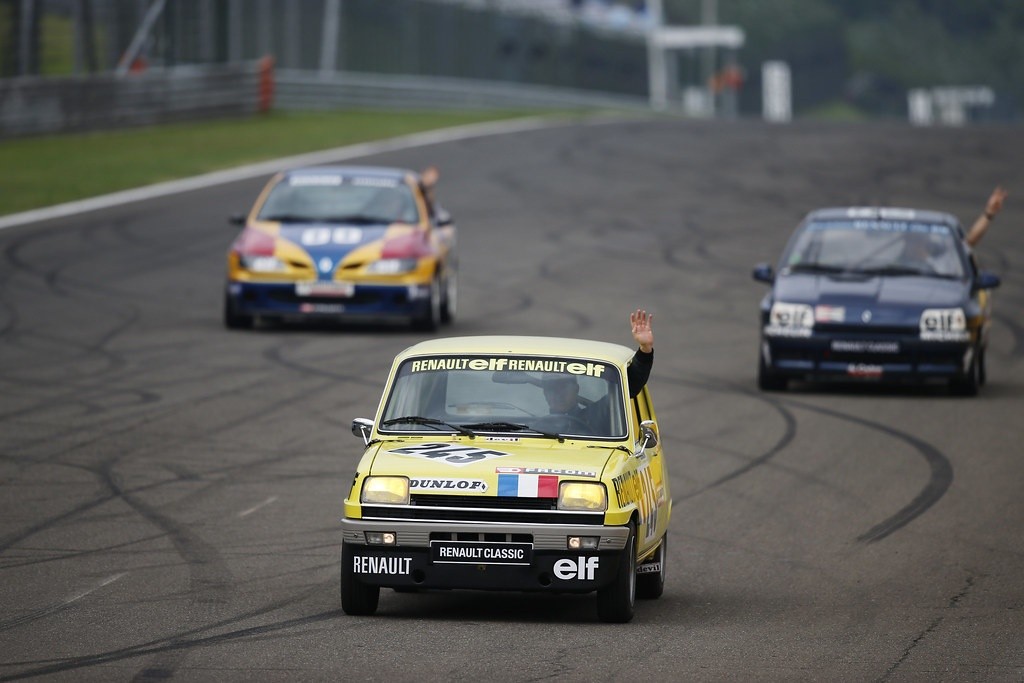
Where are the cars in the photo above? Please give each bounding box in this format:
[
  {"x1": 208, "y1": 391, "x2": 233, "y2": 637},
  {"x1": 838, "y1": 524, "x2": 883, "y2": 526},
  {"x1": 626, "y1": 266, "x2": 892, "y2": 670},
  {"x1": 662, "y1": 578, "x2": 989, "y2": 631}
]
[
  {"x1": 748, "y1": 202, "x2": 1004, "y2": 396},
  {"x1": 337, "y1": 331, "x2": 674, "y2": 621},
  {"x1": 221, "y1": 158, "x2": 459, "y2": 331}
]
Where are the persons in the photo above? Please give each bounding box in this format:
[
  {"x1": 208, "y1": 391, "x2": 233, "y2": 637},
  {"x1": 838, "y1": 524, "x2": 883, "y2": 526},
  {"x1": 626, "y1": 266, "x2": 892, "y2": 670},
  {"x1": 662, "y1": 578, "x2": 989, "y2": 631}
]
[
  {"x1": 366, "y1": 166, "x2": 439, "y2": 221},
  {"x1": 896, "y1": 184, "x2": 1007, "y2": 275},
  {"x1": 531, "y1": 307, "x2": 656, "y2": 440}
]
